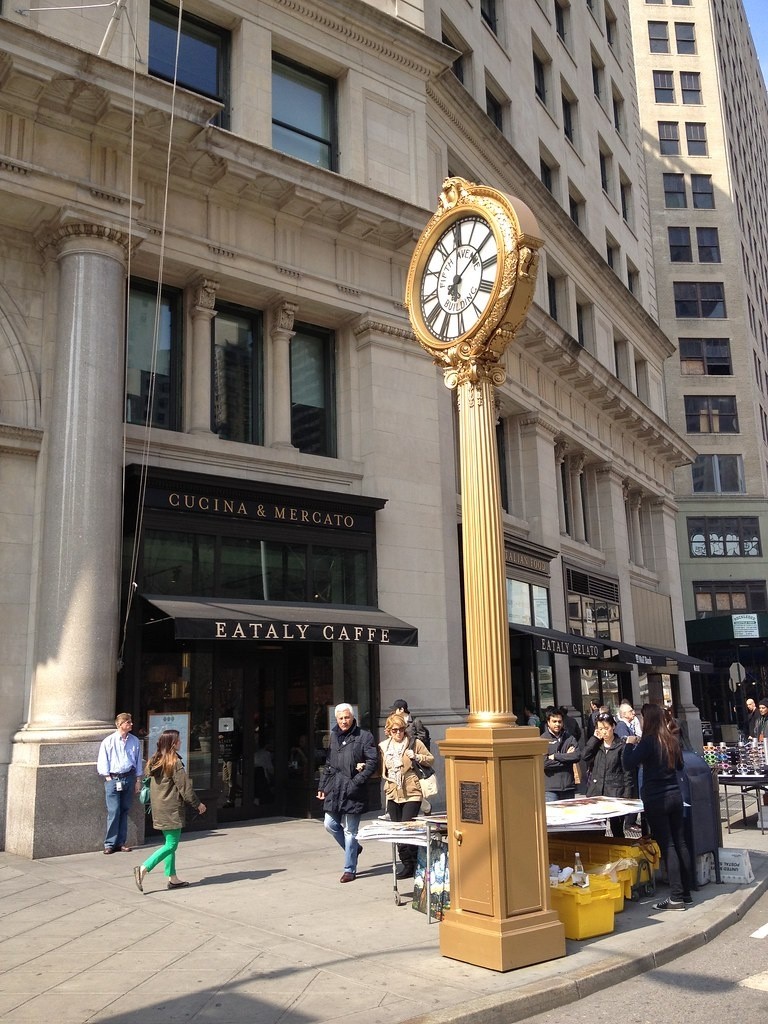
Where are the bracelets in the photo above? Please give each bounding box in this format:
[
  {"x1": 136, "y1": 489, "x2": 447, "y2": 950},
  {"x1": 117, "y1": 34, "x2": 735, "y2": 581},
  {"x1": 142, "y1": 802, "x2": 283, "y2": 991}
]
[{"x1": 136, "y1": 780, "x2": 142, "y2": 782}]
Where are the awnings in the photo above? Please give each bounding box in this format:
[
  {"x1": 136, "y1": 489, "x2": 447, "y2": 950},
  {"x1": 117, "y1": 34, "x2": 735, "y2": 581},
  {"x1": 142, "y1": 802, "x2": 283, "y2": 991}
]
[
  {"x1": 140, "y1": 592, "x2": 418, "y2": 647},
  {"x1": 508, "y1": 622, "x2": 714, "y2": 673}
]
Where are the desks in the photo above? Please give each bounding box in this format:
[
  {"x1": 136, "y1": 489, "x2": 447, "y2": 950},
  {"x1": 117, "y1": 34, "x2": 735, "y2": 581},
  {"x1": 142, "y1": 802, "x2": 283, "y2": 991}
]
[
  {"x1": 358, "y1": 794, "x2": 646, "y2": 925},
  {"x1": 717, "y1": 774, "x2": 768, "y2": 835}
]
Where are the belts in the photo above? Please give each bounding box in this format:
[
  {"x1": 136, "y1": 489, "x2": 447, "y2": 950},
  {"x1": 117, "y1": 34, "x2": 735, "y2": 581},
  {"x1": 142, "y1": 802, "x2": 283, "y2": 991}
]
[{"x1": 110, "y1": 771, "x2": 132, "y2": 778}]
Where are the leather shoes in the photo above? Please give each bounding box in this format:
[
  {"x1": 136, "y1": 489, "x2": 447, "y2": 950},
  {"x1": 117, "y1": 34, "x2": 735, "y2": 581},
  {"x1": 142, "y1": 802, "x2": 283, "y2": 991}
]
[
  {"x1": 357, "y1": 845, "x2": 363, "y2": 858},
  {"x1": 339, "y1": 871, "x2": 355, "y2": 883}
]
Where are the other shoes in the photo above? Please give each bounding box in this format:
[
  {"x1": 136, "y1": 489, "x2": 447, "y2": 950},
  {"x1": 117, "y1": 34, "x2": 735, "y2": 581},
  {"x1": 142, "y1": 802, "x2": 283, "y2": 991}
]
[
  {"x1": 168, "y1": 881, "x2": 189, "y2": 889},
  {"x1": 114, "y1": 844, "x2": 131, "y2": 852},
  {"x1": 103, "y1": 847, "x2": 113, "y2": 854},
  {"x1": 134, "y1": 866, "x2": 143, "y2": 892}
]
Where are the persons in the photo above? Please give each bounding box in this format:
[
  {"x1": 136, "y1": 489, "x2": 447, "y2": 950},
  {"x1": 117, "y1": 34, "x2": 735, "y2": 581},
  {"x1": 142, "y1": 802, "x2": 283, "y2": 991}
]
[
  {"x1": 559, "y1": 706, "x2": 581, "y2": 753},
  {"x1": 623, "y1": 703, "x2": 693, "y2": 911},
  {"x1": 221, "y1": 710, "x2": 242, "y2": 807},
  {"x1": 318, "y1": 702, "x2": 377, "y2": 883},
  {"x1": 356, "y1": 714, "x2": 434, "y2": 880},
  {"x1": 97, "y1": 713, "x2": 143, "y2": 854},
  {"x1": 539, "y1": 706, "x2": 554, "y2": 735},
  {"x1": 586, "y1": 698, "x2": 601, "y2": 738},
  {"x1": 389, "y1": 698, "x2": 430, "y2": 752},
  {"x1": 540, "y1": 709, "x2": 581, "y2": 802},
  {"x1": 746, "y1": 698, "x2": 768, "y2": 742},
  {"x1": 525, "y1": 703, "x2": 541, "y2": 727},
  {"x1": 134, "y1": 730, "x2": 206, "y2": 892},
  {"x1": 616, "y1": 699, "x2": 641, "y2": 738},
  {"x1": 595, "y1": 705, "x2": 616, "y2": 728},
  {"x1": 581, "y1": 712, "x2": 634, "y2": 838},
  {"x1": 614, "y1": 703, "x2": 642, "y2": 833},
  {"x1": 291, "y1": 735, "x2": 319, "y2": 769}
]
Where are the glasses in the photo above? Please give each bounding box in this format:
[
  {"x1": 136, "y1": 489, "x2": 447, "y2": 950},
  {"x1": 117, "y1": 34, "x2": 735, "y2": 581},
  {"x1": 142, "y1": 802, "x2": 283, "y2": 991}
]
[
  {"x1": 598, "y1": 714, "x2": 614, "y2": 724},
  {"x1": 701, "y1": 735, "x2": 768, "y2": 776},
  {"x1": 391, "y1": 726, "x2": 406, "y2": 733}
]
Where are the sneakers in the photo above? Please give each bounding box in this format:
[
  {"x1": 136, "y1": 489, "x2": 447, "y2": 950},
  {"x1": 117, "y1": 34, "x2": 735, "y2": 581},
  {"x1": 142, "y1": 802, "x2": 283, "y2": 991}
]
[
  {"x1": 652, "y1": 897, "x2": 685, "y2": 911},
  {"x1": 378, "y1": 812, "x2": 391, "y2": 821},
  {"x1": 683, "y1": 896, "x2": 694, "y2": 904}
]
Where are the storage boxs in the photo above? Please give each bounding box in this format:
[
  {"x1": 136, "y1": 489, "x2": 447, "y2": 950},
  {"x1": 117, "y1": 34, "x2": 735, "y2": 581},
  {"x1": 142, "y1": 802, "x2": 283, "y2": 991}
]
[
  {"x1": 659, "y1": 854, "x2": 713, "y2": 886},
  {"x1": 757, "y1": 806, "x2": 768, "y2": 828},
  {"x1": 549, "y1": 835, "x2": 661, "y2": 941},
  {"x1": 711, "y1": 848, "x2": 755, "y2": 884}
]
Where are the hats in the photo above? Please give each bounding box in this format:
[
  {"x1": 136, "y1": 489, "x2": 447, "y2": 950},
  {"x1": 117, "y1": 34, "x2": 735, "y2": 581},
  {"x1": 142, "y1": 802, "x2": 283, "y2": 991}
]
[
  {"x1": 758, "y1": 698, "x2": 768, "y2": 709},
  {"x1": 389, "y1": 699, "x2": 407, "y2": 708}
]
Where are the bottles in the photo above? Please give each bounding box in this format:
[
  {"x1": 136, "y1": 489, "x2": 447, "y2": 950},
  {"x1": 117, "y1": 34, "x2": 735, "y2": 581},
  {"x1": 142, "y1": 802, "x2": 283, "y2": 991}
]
[{"x1": 574, "y1": 852, "x2": 584, "y2": 875}]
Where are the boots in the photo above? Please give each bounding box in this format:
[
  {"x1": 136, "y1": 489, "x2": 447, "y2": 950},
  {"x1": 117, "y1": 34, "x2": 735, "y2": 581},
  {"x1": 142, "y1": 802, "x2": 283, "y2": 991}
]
[{"x1": 396, "y1": 851, "x2": 416, "y2": 879}]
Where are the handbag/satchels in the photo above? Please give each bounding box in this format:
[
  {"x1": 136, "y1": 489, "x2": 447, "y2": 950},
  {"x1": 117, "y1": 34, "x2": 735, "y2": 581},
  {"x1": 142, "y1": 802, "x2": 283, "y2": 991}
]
[
  {"x1": 408, "y1": 737, "x2": 438, "y2": 798},
  {"x1": 140, "y1": 776, "x2": 151, "y2": 805}
]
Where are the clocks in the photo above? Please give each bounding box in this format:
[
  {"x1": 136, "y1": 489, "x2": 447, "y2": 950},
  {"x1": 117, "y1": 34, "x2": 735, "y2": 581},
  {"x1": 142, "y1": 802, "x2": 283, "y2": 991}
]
[{"x1": 403, "y1": 196, "x2": 518, "y2": 358}]
